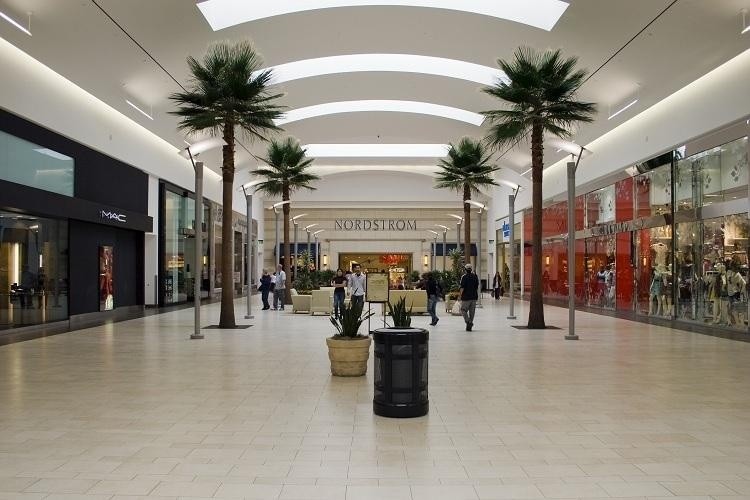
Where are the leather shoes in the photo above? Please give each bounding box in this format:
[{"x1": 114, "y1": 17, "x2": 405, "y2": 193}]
[{"x1": 430, "y1": 319, "x2": 439, "y2": 325}]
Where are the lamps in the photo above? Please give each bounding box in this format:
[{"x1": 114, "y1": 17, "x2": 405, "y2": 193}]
[
  {"x1": 545, "y1": 255, "x2": 550, "y2": 266},
  {"x1": 425, "y1": 255, "x2": 428, "y2": 266},
  {"x1": 643, "y1": 257, "x2": 647, "y2": 266},
  {"x1": 324, "y1": 255, "x2": 327, "y2": 265}
]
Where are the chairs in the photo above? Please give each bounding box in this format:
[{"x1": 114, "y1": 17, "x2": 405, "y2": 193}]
[{"x1": 290, "y1": 287, "x2": 346, "y2": 318}]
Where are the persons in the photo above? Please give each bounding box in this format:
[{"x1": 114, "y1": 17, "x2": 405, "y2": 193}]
[
  {"x1": 646, "y1": 263, "x2": 672, "y2": 315},
  {"x1": 459, "y1": 264, "x2": 479, "y2": 331},
  {"x1": 493, "y1": 272, "x2": 501, "y2": 299},
  {"x1": 395, "y1": 276, "x2": 406, "y2": 288},
  {"x1": 19, "y1": 264, "x2": 51, "y2": 311},
  {"x1": 416, "y1": 272, "x2": 439, "y2": 326},
  {"x1": 347, "y1": 265, "x2": 367, "y2": 319},
  {"x1": 679, "y1": 272, "x2": 691, "y2": 320},
  {"x1": 597, "y1": 263, "x2": 616, "y2": 308},
  {"x1": 271, "y1": 264, "x2": 286, "y2": 311},
  {"x1": 333, "y1": 269, "x2": 347, "y2": 320},
  {"x1": 258, "y1": 272, "x2": 271, "y2": 310},
  {"x1": 712, "y1": 263, "x2": 746, "y2": 326},
  {"x1": 543, "y1": 271, "x2": 550, "y2": 296},
  {"x1": 309, "y1": 264, "x2": 316, "y2": 272}
]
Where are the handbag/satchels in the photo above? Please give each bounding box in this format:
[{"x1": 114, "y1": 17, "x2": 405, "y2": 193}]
[
  {"x1": 491, "y1": 291, "x2": 494, "y2": 297},
  {"x1": 259, "y1": 285, "x2": 268, "y2": 291}
]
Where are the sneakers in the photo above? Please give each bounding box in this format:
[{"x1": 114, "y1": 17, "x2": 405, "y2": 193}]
[
  {"x1": 262, "y1": 305, "x2": 284, "y2": 310},
  {"x1": 466, "y1": 321, "x2": 473, "y2": 331}
]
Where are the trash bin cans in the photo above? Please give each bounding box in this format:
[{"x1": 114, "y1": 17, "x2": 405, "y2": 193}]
[{"x1": 373, "y1": 327, "x2": 429, "y2": 419}]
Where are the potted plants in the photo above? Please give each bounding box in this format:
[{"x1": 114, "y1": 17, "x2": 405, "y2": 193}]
[{"x1": 326, "y1": 288, "x2": 375, "y2": 376}]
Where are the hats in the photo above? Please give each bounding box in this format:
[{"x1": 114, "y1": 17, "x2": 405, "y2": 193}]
[{"x1": 465, "y1": 264, "x2": 473, "y2": 270}]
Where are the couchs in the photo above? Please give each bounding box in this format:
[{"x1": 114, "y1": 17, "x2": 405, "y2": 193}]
[{"x1": 383, "y1": 289, "x2": 428, "y2": 314}]
